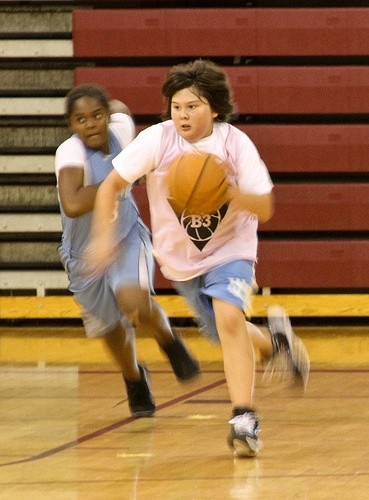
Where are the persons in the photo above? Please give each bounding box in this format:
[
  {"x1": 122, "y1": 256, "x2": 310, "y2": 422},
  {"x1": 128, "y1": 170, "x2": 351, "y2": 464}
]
[
  {"x1": 81, "y1": 60, "x2": 305, "y2": 459},
  {"x1": 54, "y1": 83, "x2": 200, "y2": 418}
]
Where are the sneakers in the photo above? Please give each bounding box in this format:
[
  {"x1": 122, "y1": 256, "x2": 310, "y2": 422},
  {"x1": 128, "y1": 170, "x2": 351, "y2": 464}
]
[
  {"x1": 273, "y1": 332, "x2": 307, "y2": 401},
  {"x1": 123, "y1": 364, "x2": 154, "y2": 418},
  {"x1": 157, "y1": 332, "x2": 199, "y2": 381},
  {"x1": 227, "y1": 416, "x2": 259, "y2": 459}
]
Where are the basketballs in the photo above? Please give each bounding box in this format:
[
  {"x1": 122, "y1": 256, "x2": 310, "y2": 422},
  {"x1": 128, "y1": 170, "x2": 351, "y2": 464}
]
[{"x1": 166, "y1": 152, "x2": 230, "y2": 216}]
